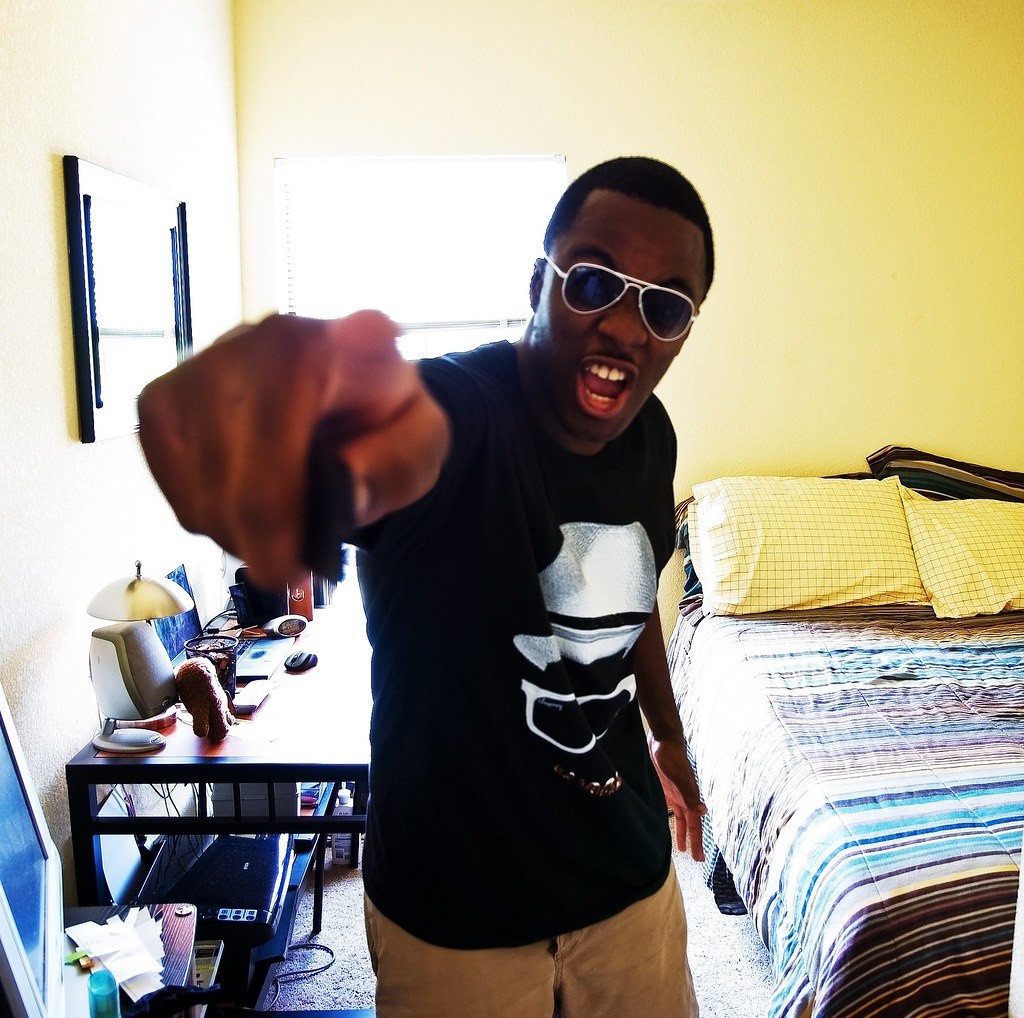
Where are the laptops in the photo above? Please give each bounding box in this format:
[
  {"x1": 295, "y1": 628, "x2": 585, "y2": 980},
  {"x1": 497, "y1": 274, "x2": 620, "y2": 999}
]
[{"x1": 143, "y1": 562, "x2": 298, "y2": 681}]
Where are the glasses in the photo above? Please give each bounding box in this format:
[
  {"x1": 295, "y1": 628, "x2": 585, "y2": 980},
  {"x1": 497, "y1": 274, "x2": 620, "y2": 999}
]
[{"x1": 544, "y1": 254, "x2": 699, "y2": 342}]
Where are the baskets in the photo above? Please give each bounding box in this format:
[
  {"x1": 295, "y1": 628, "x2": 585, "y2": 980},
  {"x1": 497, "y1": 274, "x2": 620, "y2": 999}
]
[{"x1": 184, "y1": 635, "x2": 239, "y2": 701}]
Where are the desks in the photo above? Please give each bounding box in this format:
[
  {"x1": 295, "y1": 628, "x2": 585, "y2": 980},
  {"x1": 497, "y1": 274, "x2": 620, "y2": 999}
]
[
  {"x1": 59, "y1": 903, "x2": 202, "y2": 1018},
  {"x1": 62, "y1": 561, "x2": 378, "y2": 936}
]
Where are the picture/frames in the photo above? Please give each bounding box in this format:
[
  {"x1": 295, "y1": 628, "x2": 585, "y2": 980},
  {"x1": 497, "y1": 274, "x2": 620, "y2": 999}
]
[{"x1": 60, "y1": 152, "x2": 194, "y2": 447}]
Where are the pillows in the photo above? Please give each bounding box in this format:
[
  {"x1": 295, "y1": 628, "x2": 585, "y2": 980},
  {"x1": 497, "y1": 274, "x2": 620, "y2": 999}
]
[
  {"x1": 863, "y1": 445, "x2": 1024, "y2": 505},
  {"x1": 691, "y1": 471, "x2": 933, "y2": 612},
  {"x1": 895, "y1": 482, "x2": 1024, "y2": 619},
  {"x1": 668, "y1": 472, "x2": 880, "y2": 627}
]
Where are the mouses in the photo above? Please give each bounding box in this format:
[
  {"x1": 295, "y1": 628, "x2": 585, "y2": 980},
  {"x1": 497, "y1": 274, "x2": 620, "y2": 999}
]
[{"x1": 284, "y1": 650, "x2": 318, "y2": 672}]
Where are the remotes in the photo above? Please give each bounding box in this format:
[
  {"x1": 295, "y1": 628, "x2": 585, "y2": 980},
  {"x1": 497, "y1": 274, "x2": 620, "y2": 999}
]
[{"x1": 300, "y1": 440, "x2": 358, "y2": 587}]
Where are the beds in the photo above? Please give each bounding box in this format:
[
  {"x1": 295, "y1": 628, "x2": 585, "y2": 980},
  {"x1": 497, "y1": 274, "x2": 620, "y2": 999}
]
[{"x1": 658, "y1": 444, "x2": 1024, "y2": 1018}]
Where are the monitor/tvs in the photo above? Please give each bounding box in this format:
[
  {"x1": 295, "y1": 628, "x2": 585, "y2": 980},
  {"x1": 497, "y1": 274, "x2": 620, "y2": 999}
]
[{"x1": 0, "y1": 683, "x2": 94, "y2": 1018}]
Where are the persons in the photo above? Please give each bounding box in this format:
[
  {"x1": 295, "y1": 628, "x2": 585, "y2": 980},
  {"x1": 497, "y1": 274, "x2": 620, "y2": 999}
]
[{"x1": 136, "y1": 155, "x2": 716, "y2": 1018}]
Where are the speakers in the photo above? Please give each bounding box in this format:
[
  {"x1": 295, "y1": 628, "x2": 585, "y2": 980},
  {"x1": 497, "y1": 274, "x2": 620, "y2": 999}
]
[{"x1": 90, "y1": 623, "x2": 180, "y2": 721}]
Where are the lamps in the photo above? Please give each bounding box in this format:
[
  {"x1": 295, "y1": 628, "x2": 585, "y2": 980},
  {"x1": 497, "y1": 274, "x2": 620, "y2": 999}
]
[{"x1": 84, "y1": 560, "x2": 195, "y2": 624}]
